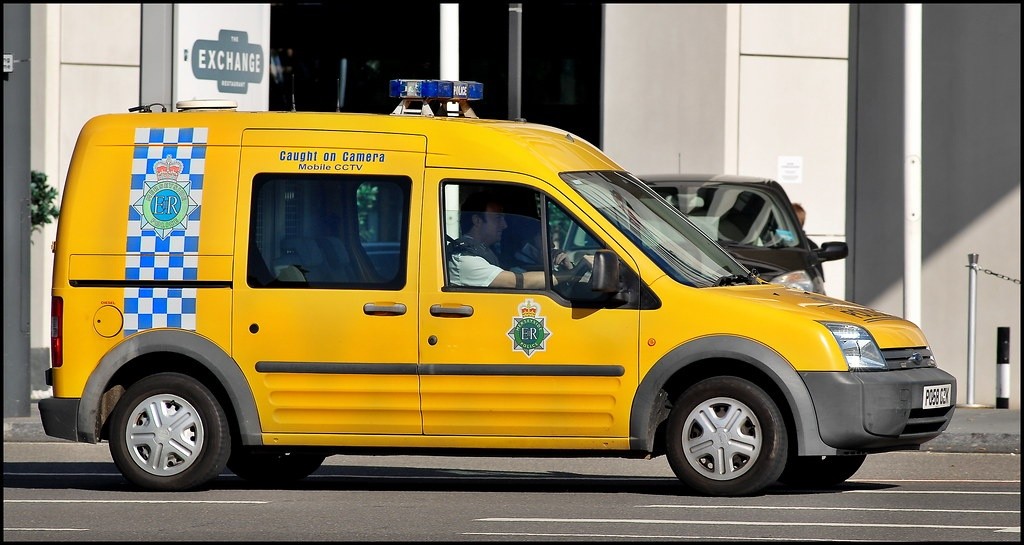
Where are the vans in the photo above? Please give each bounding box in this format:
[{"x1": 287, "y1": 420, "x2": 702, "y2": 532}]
[{"x1": 37, "y1": 79, "x2": 958, "y2": 498}]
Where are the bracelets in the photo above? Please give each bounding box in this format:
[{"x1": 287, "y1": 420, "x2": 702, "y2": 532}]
[{"x1": 571, "y1": 262, "x2": 575, "y2": 268}]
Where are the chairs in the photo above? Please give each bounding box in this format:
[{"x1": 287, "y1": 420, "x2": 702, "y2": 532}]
[
  {"x1": 348, "y1": 237, "x2": 387, "y2": 281},
  {"x1": 283, "y1": 234, "x2": 338, "y2": 282}
]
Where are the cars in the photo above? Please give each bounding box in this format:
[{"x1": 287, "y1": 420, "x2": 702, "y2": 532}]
[{"x1": 559, "y1": 174, "x2": 850, "y2": 295}]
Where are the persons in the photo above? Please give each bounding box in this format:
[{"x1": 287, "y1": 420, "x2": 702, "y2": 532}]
[
  {"x1": 447, "y1": 192, "x2": 594, "y2": 289},
  {"x1": 793, "y1": 204, "x2": 826, "y2": 293},
  {"x1": 513, "y1": 221, "x2": 575, "y2": 271}
]
